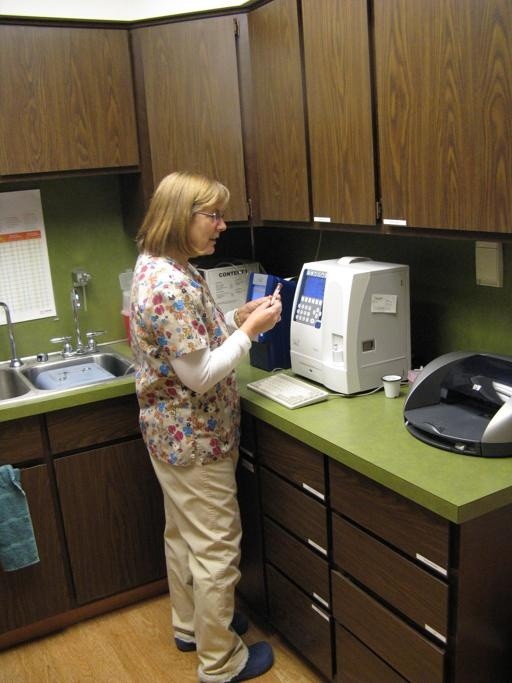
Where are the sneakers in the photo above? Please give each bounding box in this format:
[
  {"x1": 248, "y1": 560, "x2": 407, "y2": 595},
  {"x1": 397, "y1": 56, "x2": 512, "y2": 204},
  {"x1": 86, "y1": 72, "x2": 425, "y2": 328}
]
[
  {"x1": 198, "y1": 638, "x2": 274, "y2": 683},
  {"x1": 173, "y1": 610, "x2": 250, "y2": 652}
]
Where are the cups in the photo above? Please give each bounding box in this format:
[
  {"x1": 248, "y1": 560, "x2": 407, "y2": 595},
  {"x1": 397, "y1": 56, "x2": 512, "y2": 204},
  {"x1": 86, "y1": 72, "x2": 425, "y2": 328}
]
[{"x1": 382, "y1": 374, "x2": 402, "y2": 397}]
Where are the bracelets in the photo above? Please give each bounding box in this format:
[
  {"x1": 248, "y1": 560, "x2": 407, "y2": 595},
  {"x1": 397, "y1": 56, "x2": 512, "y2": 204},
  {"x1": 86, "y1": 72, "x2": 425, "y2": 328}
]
[{"x1": 234, "y1": 306, "x2": 244, "y2": 328}]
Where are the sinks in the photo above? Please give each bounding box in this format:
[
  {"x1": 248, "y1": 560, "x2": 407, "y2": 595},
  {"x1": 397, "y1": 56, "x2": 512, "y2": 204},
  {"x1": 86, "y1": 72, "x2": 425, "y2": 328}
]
[
  {"x1": 20, "y1": 345, "x2": 140, "y2": 405},
  {"x1": 0, "y1": 364, "x2": 30, "y2": 411}
]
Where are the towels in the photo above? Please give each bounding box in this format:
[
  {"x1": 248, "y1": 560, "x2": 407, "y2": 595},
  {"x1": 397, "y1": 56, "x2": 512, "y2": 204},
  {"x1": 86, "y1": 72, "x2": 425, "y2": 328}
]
[{"x1": 0, "y1": 465, "x2": 39, "y2": 573}]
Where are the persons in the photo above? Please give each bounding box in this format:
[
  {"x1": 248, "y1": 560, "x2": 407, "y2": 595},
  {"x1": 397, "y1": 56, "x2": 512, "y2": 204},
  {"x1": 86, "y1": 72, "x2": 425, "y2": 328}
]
[{"x1": 129, "y1": 172, "x2": 282, "y2": 682}]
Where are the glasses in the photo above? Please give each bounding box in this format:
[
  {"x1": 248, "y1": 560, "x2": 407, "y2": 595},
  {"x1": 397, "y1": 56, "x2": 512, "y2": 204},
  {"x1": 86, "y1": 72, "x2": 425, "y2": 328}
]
[{"x1": 193, "y1": 209, "x2": 226, "y2": 224}]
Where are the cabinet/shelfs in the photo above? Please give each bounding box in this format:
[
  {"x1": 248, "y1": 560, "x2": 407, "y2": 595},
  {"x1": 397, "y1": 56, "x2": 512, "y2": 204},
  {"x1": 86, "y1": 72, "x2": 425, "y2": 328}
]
[
  {"x1": 132, "y1": 10, "x2": 259, "y2": 229},
  {"x1": 0, "y1": 396, "x2": 165, "y2": 653},
  {"x1": 247, "y1": 0, "x2": 379, "y2": 235},
  {"x1": 2, "y1": 20, "x2": 142, "y2": 183},
  {"x1": 373, "y1": 0, "x2": 510, "y2": 242},
  {"x1": 239, "y1": 350, "x2": 512, "y2": 683}
]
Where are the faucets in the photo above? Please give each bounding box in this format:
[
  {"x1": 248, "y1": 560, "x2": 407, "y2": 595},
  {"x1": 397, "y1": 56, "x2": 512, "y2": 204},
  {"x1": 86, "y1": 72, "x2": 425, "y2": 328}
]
[
  {"x1": 49, "y1": 288, "x2": 108, "y2": 358},
  {"x1": 0, "y1": 301, "x2": 24, "y2": 367}
]
